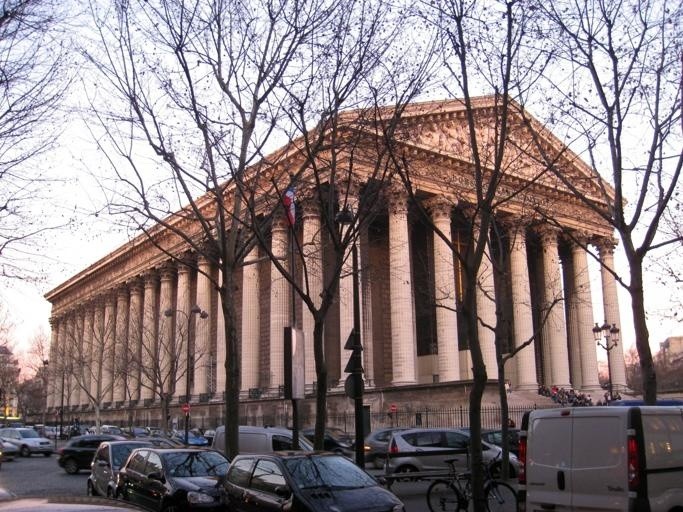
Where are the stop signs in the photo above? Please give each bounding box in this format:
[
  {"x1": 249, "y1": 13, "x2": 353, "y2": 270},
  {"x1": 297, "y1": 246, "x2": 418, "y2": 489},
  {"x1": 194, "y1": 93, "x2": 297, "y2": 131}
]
[
  {"x1": 181, "y1": 404, "x2": 192, "y2": 414},
  {"x1": 389, "y1": 405, "x2": 398, "y2": 412}
]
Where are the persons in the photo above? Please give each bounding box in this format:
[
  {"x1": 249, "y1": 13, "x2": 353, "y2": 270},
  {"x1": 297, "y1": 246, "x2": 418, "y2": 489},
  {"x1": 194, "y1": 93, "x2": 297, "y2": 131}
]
[
  {"x1": 535, "y1": 380, "x2": 621, "y2": 408},
  {"x1": 505, "y1": 381, "x2": 509, "y2": 394},
  {"x1": 508, "y1": 381, "x2": 513, "y2": 393}
]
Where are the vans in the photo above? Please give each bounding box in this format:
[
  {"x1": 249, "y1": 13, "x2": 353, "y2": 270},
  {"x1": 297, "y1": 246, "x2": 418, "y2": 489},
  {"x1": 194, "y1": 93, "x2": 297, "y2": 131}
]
[{"x1": 211, "y1": 423, "x2": 354, "y2": 454}]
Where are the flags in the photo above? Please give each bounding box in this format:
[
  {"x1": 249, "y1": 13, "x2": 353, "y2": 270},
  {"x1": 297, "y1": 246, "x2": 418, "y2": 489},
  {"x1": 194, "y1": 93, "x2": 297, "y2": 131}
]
[{"x1": 281, "y1": 173, "x2": 296, "y2": 227}]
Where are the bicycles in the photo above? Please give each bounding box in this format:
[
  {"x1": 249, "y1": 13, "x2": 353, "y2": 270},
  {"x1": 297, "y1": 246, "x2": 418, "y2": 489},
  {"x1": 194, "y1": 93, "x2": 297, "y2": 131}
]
[{"x1": 425, "y1": 451, "x2": 519, "y2": 510}]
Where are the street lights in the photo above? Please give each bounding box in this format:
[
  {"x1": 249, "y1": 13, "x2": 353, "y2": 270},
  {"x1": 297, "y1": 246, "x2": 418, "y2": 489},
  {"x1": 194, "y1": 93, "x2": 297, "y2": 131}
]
[
  {"x1": 332, "y1": 201, "x2": 364, "y2": 469},
  {"x1": 164, "y1": 304, "x2": 208, "y2": 446},
  {"x1": 593, "y1": 320, "x2": 620, "y2": 400}
]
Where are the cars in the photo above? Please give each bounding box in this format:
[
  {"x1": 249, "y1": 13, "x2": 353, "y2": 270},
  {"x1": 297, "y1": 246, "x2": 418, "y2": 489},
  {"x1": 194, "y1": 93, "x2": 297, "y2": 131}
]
[
  {"x1": 54, "y1": 423, "x2": 230, "y2": 511},
  {"x1": 224, "y1": 451, "x2": 406, "y2": 511},
  {"x1": 1, "y1": 423, "x2": 56, "y2": 458},
  {"x1": 356, "y1": 427, "x2": 524, "y2": 481}
]
